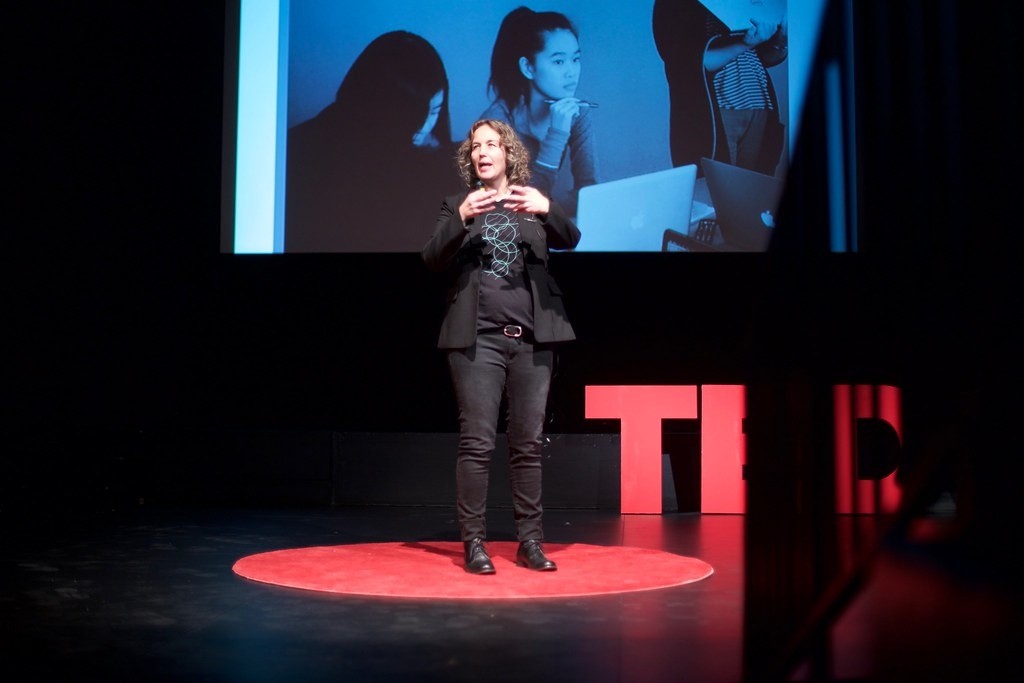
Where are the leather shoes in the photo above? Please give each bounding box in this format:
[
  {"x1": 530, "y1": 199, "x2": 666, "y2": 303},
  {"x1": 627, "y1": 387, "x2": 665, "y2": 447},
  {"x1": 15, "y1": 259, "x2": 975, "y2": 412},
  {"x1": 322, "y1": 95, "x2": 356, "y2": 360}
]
[
  {"x1": 464, "y1": 538, "x2": 495, "y2": 574},
  {"x1": 517, "y1": 540, "x2": 558, "y2": 571}
]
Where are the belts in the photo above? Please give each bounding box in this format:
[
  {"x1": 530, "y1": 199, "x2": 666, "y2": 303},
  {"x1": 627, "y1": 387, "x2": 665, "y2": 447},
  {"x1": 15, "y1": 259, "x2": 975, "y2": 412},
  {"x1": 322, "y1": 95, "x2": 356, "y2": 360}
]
[{"x1": 477, "y1": 324, "x2": 527, "y2": 338}]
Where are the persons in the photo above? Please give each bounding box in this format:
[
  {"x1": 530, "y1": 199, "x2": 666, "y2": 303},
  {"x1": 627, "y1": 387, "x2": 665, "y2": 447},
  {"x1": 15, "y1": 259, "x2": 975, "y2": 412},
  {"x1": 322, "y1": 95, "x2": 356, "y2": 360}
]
[
  {"x1": 416, "y1": 118, "x2": 582, "y2": 575},
  {"x1": 468, "y1": 5, "x2": 599, "y2": 202},
  {"x1": 284, "y1": 28, "x2": 468, "y2": 250},
  {"x1": 653, "y1": 0, "x2": 787, "y2": 178}
]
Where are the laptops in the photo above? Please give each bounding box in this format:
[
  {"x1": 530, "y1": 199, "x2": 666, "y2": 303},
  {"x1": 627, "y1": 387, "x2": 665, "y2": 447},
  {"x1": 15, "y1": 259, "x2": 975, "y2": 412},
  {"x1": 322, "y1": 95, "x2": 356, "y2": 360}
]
[
  {"x1": 701, "y1": 157, "x2": 779, "y2": 253},
  {"x1": 698, "y1": 0, "x2": 787, "y2": 48}
]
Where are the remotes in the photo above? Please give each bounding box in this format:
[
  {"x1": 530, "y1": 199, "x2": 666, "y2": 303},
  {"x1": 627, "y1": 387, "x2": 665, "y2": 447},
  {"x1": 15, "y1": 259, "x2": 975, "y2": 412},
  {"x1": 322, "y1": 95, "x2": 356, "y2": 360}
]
[{"x1": 476, "y1": 182, "x2": 489, "y2": 191}]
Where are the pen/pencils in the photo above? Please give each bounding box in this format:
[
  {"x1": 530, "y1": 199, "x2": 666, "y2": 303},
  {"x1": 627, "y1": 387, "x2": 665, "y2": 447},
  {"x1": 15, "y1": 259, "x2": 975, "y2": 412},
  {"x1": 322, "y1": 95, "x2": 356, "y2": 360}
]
[{"x1": 543, "y1": 99, "x2": 599, "y2": 108}]
